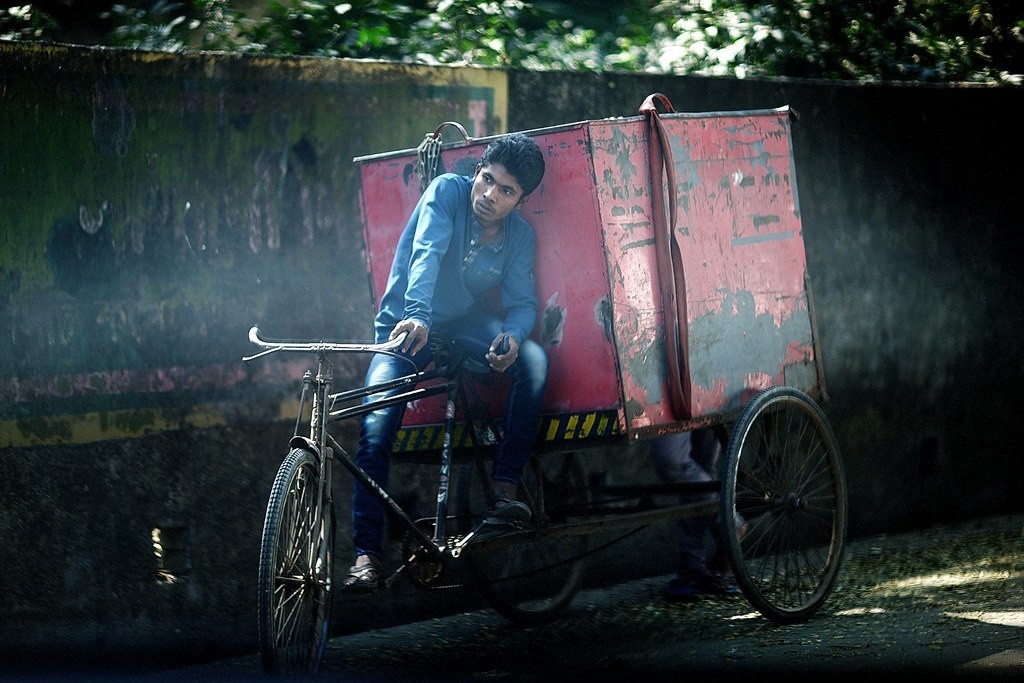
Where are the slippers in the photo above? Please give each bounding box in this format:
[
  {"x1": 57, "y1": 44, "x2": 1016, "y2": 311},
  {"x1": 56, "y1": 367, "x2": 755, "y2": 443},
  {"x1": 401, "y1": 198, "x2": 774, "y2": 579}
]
[
  {"x1": 343, "y1": 561, "x2": 387, "y2": 589},
  {"x1": 488, "y1": 495, "x2": 532, "y2": 521}
]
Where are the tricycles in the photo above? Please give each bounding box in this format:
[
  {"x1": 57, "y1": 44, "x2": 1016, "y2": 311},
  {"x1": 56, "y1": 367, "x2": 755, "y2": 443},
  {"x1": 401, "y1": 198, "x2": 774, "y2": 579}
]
[{"x1": 248, "y1": 99, "x2": 848, "y2": 680}]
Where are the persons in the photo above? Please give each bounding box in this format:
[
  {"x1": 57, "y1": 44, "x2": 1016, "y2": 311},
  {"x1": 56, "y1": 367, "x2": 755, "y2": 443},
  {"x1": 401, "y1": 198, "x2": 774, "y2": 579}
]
[
  {"x1": 647, "y1": 431, "x2": 748, "y2": 601},
  {"x1": 341, "y1": 134, "x2": 550, "y2": 591}
]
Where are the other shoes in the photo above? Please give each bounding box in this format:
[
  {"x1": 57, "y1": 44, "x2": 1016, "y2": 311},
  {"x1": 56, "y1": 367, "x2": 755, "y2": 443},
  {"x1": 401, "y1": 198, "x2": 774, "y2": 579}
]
[{"x1": 675, "y1": 520, "x2": 750, "y2": 596}]
